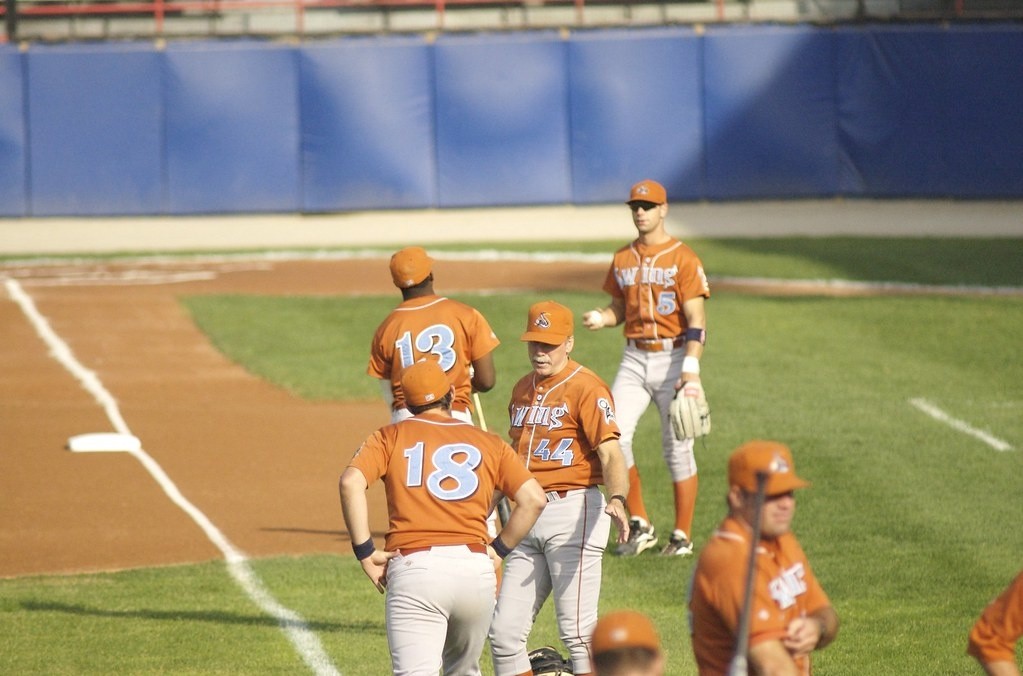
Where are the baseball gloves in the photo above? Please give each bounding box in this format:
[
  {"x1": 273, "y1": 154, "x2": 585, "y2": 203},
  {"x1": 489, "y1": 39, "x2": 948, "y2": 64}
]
[{"x1": 669, "y1": 373, "x2": 711, "y2": 439}]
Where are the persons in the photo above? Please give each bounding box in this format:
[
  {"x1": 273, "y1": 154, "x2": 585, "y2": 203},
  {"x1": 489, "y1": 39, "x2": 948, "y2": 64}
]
[
  {"x1": 688, "y1": 441, "x2": 840, "y2": 676},
  {"x1": 339, "y1": 359, "x2": 546, "y2": 676},
  {"x1": 965, "y1": 569, "x2": 1023, "y2": 676},
  {"x1": 590, "y1": 609, "x2": 665, "y2": 676},
  {"x1": 582, "y1": 179, "x2": 710, "y2": 558},
  {"x1": 365, "y1": 246, "x2": 503, "y2": 598},
  {"x1": 479, "y1": 302, "x2": 630, "y2": 676}
]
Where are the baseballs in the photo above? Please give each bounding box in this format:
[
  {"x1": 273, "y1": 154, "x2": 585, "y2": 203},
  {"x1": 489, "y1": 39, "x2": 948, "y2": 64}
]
[{"x1": 588, "y1": 311, "x2": 602, "y2": 325}]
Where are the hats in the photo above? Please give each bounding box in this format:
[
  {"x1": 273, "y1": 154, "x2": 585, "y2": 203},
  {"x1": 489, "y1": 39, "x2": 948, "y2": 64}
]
[
  {"x1": 520, "y1": 300, "x2": 574, "y2": 345},
  {"x1": 591, "y1": 611, "x2": 661, "y2": 655},
  {"x1": 400, "y1": 360, "x2": 450, "y2": 406},
  {"x1": 626, "y1": 180, "x2": 667, "y2": 205},
  {"x1": 390, "y1": 246, "x2": 435, "y2": 288},
  {"x1": 728, "y1": 439, "x2": 807, "y2": 497}
]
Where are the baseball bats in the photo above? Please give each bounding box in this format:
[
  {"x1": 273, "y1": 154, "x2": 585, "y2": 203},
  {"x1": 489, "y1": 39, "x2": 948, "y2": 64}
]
[
  {"x1": 472, "y1": 390, "x2": 511, "y2": 528},
  {"x1": 730, "y1": 470, "x2": 772, "y2": 676}
]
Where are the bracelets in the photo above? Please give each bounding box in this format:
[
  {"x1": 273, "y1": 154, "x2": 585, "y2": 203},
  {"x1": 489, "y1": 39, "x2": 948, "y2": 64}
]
[
  {"x1": 490, "y1": 535, "x2": 514, "y2": 560},
  {"x1": 682, "y1": 357, "x2": 701, "y2": 375},
  {"x1": 611, "y1": 495, "x2": 627, "y2": 509},
  {"x1": 351, "y1": 536, "x2": 376, "y2": 560}
]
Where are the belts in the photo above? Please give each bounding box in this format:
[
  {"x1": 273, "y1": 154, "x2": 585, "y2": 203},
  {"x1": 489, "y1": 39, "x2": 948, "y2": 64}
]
[
  {"x1": 400, "y1": 542, "x2": 488, "y2": 557},
  {"x1": 627, "y1": 337, "x2": 684, "y2": 351},
  {"x1": 547, "y1": 484, "x2": 599, "y2": 503}
]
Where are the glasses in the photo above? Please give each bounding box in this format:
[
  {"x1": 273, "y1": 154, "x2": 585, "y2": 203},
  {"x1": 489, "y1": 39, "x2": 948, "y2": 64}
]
[{"x1": 630, "y1": 202, "x2": 659, "y2": 211}]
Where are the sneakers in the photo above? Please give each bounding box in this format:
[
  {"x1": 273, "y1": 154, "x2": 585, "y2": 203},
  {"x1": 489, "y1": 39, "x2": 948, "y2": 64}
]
[
  {"x1": 660, "y1": 529, "x2": 693, "y2": 555},
  {"x1": 614, "y1": 515, "x2": 656, "y2": 557}
]
